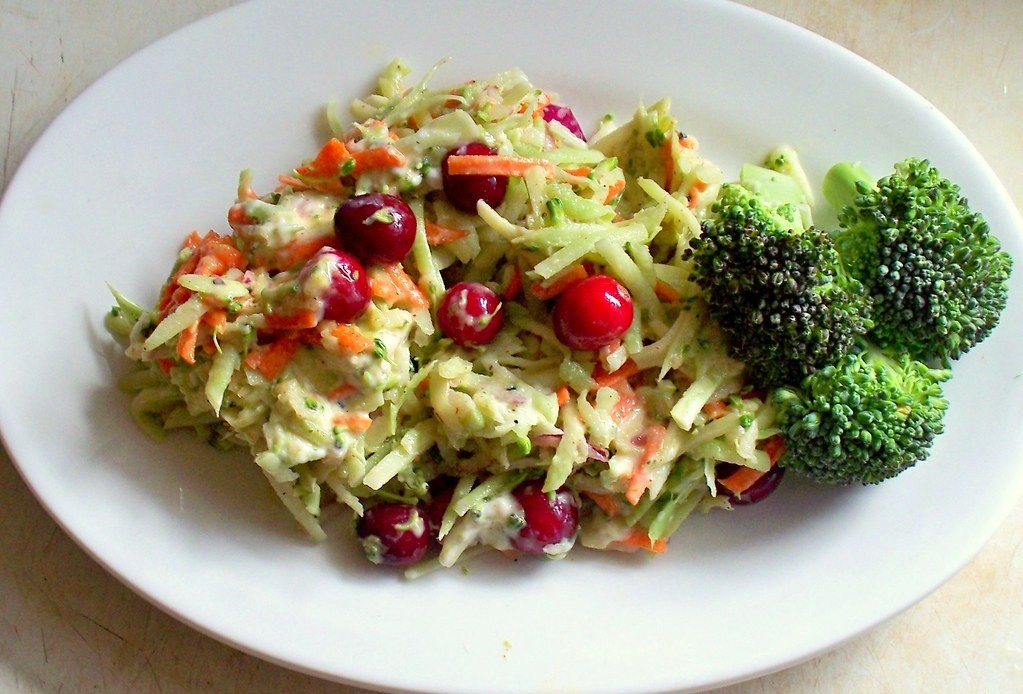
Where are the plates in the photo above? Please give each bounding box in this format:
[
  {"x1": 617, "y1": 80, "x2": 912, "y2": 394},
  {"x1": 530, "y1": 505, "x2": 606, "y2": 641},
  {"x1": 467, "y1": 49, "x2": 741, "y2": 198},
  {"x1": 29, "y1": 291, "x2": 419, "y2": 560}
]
[{"x1": 1, "y1": 1, "x2": 1023, "y2": 693}]
